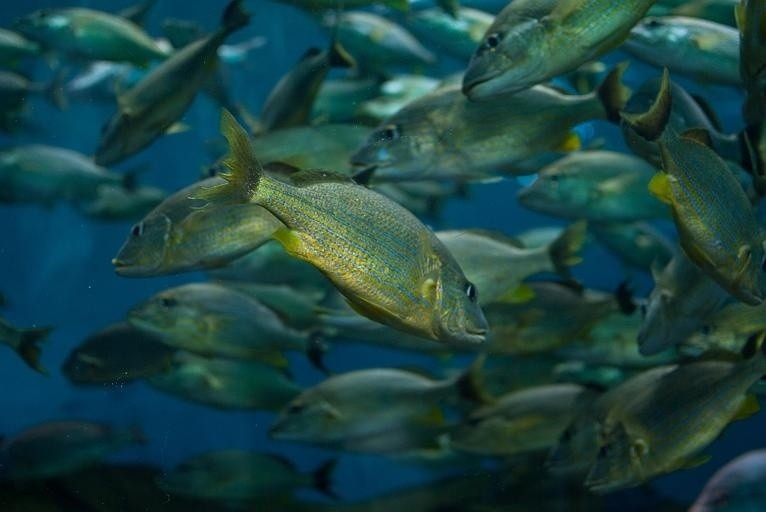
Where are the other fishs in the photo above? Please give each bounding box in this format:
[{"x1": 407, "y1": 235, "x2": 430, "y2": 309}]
[{"x1": 0, "y1": 0, "x2": 766, "y2": 512}]
